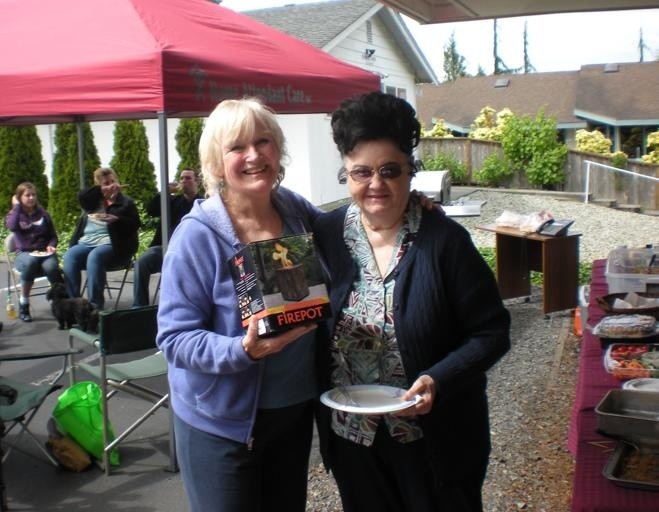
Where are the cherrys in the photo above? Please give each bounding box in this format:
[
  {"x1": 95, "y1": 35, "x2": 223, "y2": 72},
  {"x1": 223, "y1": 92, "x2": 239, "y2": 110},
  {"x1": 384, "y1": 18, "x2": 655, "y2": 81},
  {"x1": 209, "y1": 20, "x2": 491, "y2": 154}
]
[{"x1": 610, "y1": 345, "x2": 647, "y2": 359}]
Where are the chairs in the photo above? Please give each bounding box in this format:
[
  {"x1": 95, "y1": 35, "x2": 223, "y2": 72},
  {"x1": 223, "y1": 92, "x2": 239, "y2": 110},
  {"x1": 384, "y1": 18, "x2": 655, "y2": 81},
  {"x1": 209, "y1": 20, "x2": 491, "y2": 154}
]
[
  {"x1": 1, "y1": 347, "x2": 86, "y2": 469},
  {"x1": 2, "y1": 233, "x2": 66, "y2": 317},
  {"x1": 148, "y1": 225, "x2": 172, "y2": 306},
  {"x1": 67, "y1": 307, "x2": 168, "y2": 476},
  {"x1": 68, "y1": 226, "x2": 139, "y2": 310}
]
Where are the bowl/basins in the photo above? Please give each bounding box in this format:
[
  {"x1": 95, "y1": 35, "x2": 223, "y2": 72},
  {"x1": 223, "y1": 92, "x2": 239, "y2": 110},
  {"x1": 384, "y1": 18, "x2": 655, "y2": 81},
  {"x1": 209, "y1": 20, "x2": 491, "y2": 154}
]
[{"x1": 593, "y1": 291, "x2": 659, "y2": 314}]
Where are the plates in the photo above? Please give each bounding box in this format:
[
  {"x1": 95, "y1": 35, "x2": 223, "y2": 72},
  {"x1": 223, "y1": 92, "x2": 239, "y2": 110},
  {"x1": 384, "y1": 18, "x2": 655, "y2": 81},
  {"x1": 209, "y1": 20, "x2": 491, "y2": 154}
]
[
  {"x1": 28, "y1": 251, "x2": 53, "y2": 258},
  {"x1": 320, "y1": 384, "x2": 423, "y2": 414},
  {"x1": 86, "y1": 213, "x2": 118, "y2": 221},
  {"x1": 619, "y1": 378, "x2": 658, "y2": 391},
  {"x1": 589, "y1": 316, "x2": 658, "y2": 340}
]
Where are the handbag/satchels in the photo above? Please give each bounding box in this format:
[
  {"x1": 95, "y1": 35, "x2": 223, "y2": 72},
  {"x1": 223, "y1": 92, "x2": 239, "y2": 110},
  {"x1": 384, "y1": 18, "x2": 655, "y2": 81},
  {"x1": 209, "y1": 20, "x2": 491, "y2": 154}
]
[
  {"x1": 46, "y1": 434, "x2": 91, "y2": 471},
  {"x1": 53, "y1": 381, "x2": 120, "y2": 465}
]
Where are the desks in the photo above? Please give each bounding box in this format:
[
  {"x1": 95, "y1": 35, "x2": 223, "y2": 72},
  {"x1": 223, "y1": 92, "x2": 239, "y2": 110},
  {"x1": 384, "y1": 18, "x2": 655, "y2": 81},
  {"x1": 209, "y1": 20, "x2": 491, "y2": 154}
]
[
  {"x1": 563, "y1": 254, "x2": 659, "y2": 512},
  {"x1": 472, "y1": 222, "x2": 584, "y2": 321}
]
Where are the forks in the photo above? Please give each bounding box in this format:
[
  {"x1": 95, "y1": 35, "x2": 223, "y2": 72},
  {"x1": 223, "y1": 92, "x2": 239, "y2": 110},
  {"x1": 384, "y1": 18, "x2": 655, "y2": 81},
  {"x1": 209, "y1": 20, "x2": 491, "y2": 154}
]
[{"x1": 335, "y1": 380, "x2": 358, "y2": 407}]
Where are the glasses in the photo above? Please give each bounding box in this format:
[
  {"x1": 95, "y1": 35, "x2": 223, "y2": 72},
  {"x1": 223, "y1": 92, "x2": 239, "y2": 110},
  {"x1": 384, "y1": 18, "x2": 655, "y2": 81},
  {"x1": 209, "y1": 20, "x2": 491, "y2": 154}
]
[{"x1": 346, "y1": 162, "x2": 410, "y2": 182}]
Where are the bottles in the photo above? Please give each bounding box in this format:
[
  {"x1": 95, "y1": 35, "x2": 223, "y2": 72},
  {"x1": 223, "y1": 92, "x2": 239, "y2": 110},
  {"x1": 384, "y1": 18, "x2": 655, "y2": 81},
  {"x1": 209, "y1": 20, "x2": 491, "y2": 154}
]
[{"x1": 5, "y1": 293, "x2": 16, "y2": 320}]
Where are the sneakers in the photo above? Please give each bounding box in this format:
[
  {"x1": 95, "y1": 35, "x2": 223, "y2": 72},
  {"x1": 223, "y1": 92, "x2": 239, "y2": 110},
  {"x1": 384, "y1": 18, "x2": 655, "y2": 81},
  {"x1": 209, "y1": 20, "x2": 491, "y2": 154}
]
[{"x1": 19, "y1": 301, "x2": 33, "y2": 321}]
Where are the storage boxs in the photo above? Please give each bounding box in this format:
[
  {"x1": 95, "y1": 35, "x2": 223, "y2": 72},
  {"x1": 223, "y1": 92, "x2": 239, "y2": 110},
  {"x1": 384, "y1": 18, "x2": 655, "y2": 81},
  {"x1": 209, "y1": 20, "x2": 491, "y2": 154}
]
[{"x1": 226, "y1": 231, "x2": 336, "y2": 341}]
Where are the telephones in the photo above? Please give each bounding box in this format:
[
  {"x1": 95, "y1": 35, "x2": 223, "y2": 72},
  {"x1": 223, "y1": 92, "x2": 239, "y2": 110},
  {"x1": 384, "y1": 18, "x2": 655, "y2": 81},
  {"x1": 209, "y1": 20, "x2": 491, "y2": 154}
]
[{"x1": 536, "y1": 219, "x2": 575, "y2": 236}]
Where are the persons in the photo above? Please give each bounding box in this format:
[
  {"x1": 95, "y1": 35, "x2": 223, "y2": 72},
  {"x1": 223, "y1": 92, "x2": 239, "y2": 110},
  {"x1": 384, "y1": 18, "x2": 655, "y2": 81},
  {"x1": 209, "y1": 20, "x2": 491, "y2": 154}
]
[
  {"x1": 6, "y1": 181, "x2": 64, "y2": 322},
  {"x1": 157, "y1": 97, "x2": 446, "y2": 512},
  {"x1": 131, "y1": 167, "x2": 205, "y2": 311},
  {"x1": 62, "y1": 166, "x2": 140, "y2": 311},
  {"x1": 309, "y1": 90, "x2": 512, "y2": 512}
]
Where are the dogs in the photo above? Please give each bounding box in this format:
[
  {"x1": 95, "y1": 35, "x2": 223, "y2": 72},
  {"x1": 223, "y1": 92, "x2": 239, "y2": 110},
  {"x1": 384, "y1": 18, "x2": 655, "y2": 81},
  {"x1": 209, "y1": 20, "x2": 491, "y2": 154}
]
[{"x1": 47, "y1": 283, "x2": 99, "y2": 331}]
